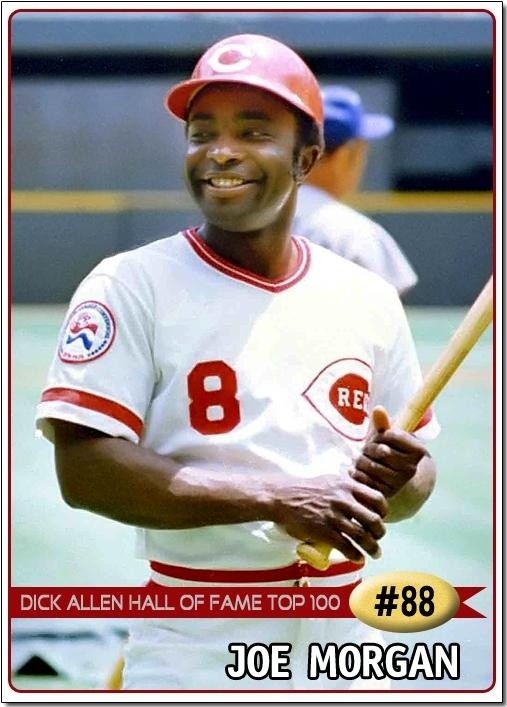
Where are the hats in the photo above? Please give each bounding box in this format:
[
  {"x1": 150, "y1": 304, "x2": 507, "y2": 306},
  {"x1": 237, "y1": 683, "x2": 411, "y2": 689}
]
[{"x1": 319, "y1": 84, "x2": 395, "y2": 154}]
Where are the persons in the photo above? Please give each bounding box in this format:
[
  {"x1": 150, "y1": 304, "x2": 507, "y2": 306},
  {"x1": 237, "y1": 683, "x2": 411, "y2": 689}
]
[
  {"x1": 289, "y1": 86, "x2": 420, "y2": 295},
  {"x1": 35, "y1": 34, "x2": 442, "y2": 690}
]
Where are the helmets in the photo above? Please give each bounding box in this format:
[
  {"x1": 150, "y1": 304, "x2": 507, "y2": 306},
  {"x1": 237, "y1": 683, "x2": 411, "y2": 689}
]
[{"x1": 165, "y1": 33, "x2": 324, "y2": 130}]
